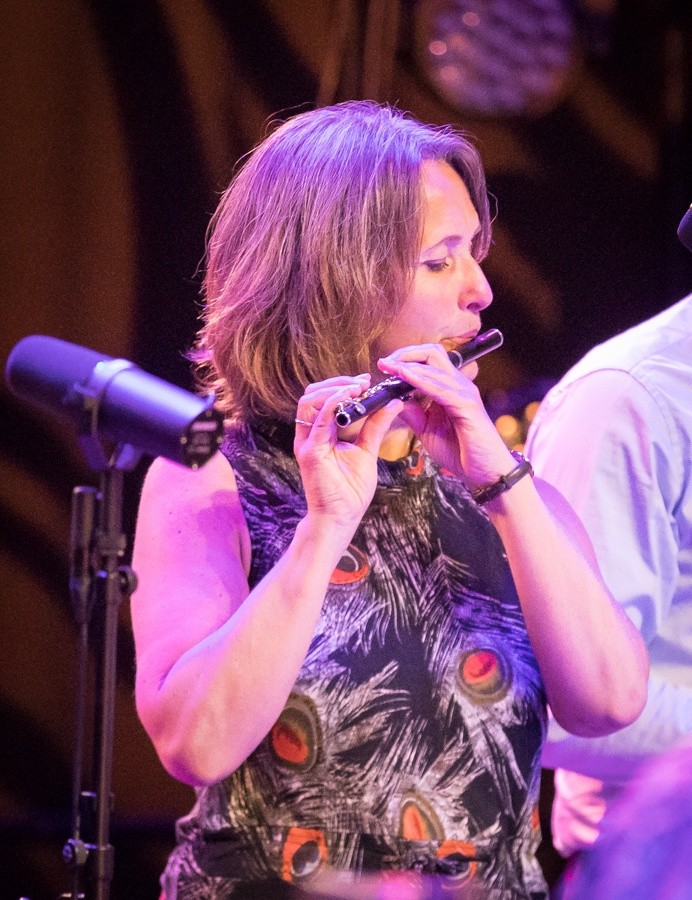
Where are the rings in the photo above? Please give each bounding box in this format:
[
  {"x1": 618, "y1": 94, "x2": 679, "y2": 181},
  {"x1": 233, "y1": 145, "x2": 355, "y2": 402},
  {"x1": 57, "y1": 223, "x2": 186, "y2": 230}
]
[{"x1": 295, "y1": 417, "x2": 313, "y2": 427}]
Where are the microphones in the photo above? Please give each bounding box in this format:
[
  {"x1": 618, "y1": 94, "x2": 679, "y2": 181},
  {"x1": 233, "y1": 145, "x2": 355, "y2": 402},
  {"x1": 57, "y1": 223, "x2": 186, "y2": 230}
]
[{"x1": 4, "y1": 333, "x2": 223, "y2": 471}]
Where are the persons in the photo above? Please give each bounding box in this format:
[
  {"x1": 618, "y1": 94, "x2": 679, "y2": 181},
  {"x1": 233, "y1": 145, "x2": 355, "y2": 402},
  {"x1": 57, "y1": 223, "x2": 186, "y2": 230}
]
[
  {"x1": 130, "y1": 100, "x2": 647, "y2": 900},
  {"x1": 523, "y1": 200, "x2": 692, "y2": 860}
]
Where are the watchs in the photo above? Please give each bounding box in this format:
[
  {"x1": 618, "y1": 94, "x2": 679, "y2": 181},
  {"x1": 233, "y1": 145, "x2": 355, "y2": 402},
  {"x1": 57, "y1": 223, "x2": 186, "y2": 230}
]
[{"x1": 470, "y1": 450, "x2": 535, "y2": 507}]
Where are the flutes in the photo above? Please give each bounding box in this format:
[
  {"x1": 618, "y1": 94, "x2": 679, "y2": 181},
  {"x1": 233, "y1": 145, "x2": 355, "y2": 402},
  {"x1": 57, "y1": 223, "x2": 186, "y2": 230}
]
[{"x1": 335, "y1": 326, "x2": 505, "y2": 430}]
